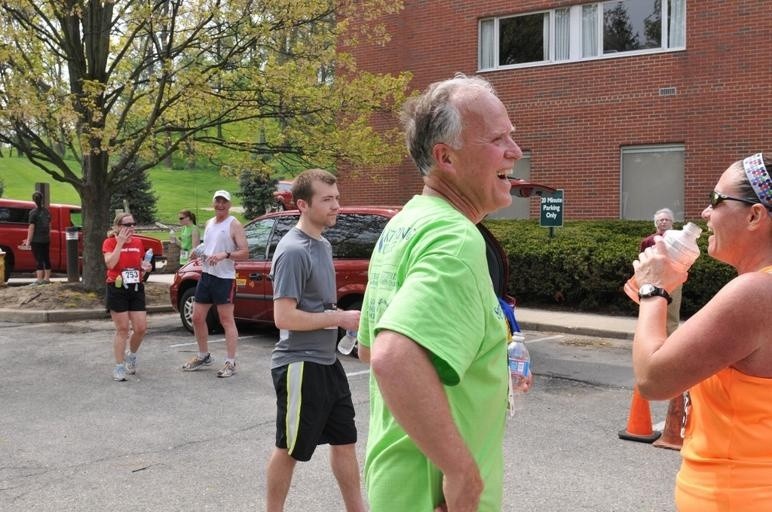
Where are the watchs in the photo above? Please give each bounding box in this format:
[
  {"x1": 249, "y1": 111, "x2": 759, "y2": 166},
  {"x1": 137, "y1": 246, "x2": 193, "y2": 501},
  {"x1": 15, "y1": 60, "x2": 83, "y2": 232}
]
[{"x1": 637, "y1": 284, "x2": 674, "y2": 306}]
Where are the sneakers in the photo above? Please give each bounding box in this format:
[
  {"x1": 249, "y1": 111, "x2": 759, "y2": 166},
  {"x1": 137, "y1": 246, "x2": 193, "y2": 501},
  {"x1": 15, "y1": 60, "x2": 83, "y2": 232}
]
[
  {"x1": 41, "y1": 280, "x2": 50, "y2": 284},
  {"x1": 123, "y1": 350, "x2": 137, "y2": 375},
  {"x1": 182, "y1": 352, "x2": 215, "y2": 372},
  {"x1": 112, "y1": 365, "x2": 128, "y2": 381},
  {"x1": 216, "y1": 360, "x2": 238, "y2": 378},
  {"x1": 29, "y1": 280, "x2": 42, "y2": 285}
]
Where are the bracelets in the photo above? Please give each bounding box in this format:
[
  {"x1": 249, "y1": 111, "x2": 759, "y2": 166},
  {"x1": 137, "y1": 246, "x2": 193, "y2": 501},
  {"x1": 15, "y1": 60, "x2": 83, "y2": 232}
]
[{"x1": 224, "y1": 249, "x2": 231, "y2": 259}]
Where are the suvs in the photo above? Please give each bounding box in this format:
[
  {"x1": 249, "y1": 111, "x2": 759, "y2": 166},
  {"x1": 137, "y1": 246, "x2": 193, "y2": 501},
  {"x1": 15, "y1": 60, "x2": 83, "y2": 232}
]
[
  {"x1": 0, "y1": 199, "x2": 167, "y2": 293},
  {"x1": 168, "y1": 179, "x2": 558, "y2": 342}
]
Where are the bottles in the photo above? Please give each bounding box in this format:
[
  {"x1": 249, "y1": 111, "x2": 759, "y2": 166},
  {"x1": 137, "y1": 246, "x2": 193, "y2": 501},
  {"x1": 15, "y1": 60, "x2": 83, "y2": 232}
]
[
  {"x1": 200, "y1": 243, "x2": 209, "y2": 272},
  {"x1": 625, "y1": 222, "x2": 703, "y2": 306},
  {"x1": 507, "y1": 331, "x2": 530, "y2": 410},
  {"x1": 338, "y1": 329, "x2": 358, "y2": 355},
  {"x1": 141, "y1": 248, "x2": 153, "y2": 270}
]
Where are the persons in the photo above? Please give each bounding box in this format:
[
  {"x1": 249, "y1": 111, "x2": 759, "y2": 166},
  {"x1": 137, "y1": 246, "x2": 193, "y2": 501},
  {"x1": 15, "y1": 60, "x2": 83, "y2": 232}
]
[
  {"x1": 23, "y1": 191, "x2": 52, "y2": 285},
  {"x1": 359, "y1": 76, "x2": 533, "y2": 511},
  {"x1": 169, "y1": 210, "x2": 200, "y2": 273},
  {"x1": 264, "y1": 169, "x2": 364, "y2": 510},
  {"x1": 183, "y1": 190, "x2": 250, "y2": 377},
  {"x1": 641, "y1": 208, "x2": 684, "y2": 337},
  {"x1": 101, "y1": 212, "x2": 152, "y2": 381},
  {"x1": 631, "y1": 152, "x2": 772, "y2": 511}
]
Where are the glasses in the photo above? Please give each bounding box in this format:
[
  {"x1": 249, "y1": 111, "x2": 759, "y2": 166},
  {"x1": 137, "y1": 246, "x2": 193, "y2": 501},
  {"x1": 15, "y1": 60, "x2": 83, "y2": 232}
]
[
  {"x1": 119, "y1": 222, "x2": 136, "y2": 226},
  {"x1": 179, "y1": 217, "x2": 186, "y2": 220},
  {"x1": 710, "y1": 191, "x2": 756, "y2": 209}
]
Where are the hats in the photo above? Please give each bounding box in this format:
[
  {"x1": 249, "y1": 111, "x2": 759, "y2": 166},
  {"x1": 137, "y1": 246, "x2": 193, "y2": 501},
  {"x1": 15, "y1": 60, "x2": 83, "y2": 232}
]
[{"x1": 213, "y1": 190, "x2": 231, "y2": 201}]
